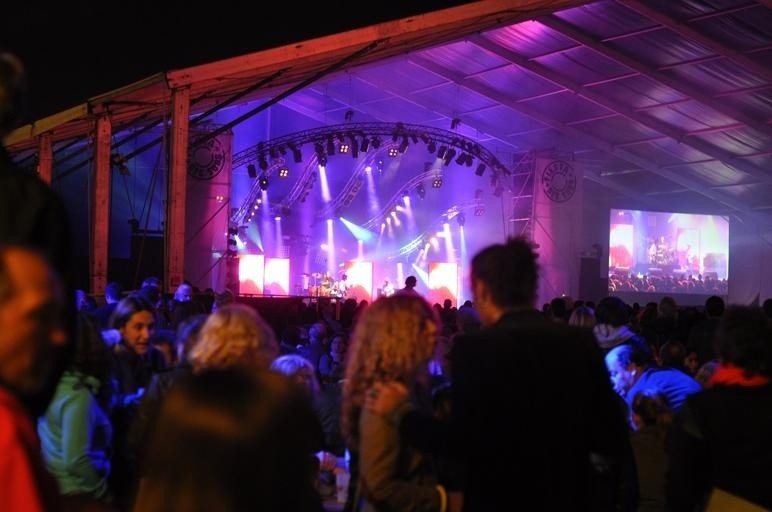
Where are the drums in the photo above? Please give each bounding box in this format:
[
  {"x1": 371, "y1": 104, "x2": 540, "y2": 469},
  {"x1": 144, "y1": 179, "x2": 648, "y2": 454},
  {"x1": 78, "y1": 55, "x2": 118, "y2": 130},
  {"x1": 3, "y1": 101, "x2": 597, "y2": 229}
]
[
  {"x1": 319, "y1": 286, "x2": 330, "y2": 297},
  {"x1": 308, "y1": 285, "x2": 320, "y2": 297}
]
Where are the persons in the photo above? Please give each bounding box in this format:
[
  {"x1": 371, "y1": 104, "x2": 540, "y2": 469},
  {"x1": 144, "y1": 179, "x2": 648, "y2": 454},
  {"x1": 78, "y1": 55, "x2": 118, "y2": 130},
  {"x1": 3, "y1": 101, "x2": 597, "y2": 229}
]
[
  {"x1": 574, "y1": 300, "x2": 583, "y2": 310},
  {"x1": 342, "y1": 296, "x2": 438, "y2": 511},
  {"x1": 205, "y1": 288, "x2": 234, "y2": 315},
  {"x1": 309, "y1": 324, "x2": 330, "y2": 368},
  {"x1": 126, "y1": 315, "x2": 209, "y2": 450},
  {"x1": 98, "y1": 282, "x2": 122, "y2": 324},
  {"x1": 433, "y1": 298, "x2": 473, "y2": 334},
  {"x1": 561, "y1": 296, "x2": 572, "y2": 311},
  {"x1": 135, "y1": 305, "x2": 327, "y2": 512},
  {"x1": 656, "y1": 297, "x2": 689, "y2": 337},
  {"x1": 318, "y1": 335, "x2": 347, "y2": 389},
  {"x1": 0, "y1": 249, "x2": 67, "y2": 512},
  {"x1": 697, "y1": 298, "x2": 727, "y2": 351},
  {"x1": 271, "y1": 355, "x2": 314, "y2": 398},
  {"x1": 137, "y1": 278, "x2": 163, "y2": 309},
  {"x1": 679, "y1": 349, "x2": 699, "y2": 380},
  {"x1": 569, "y1": 306, "x2": 596, "y2": 329},
  {"x1": 630, "y1": 302, "x2": 656, "y2": 331},
  {"x1": 35, "y1": 332, "x2": 124, "y2": 512},
  {"x1": 401, "y1": 243, "x2": 641, "y2": 512},
  {"x1": 585, "y1": 302, "x2": 595, "y2": 310},
  {"x1": 764, "y1": 299, "x2": 771, "y2": 316},
  {"x1": 606, "y1": 271, "x2": 727, "y2": 296},
  {"x1": 604, "y1": 344, "x2": 702, "y2": 434},
  {"x1": 342, "y1": 298, "x2": 368, "y2": 329},
  {"x1": 550, "y1": 298, "x2": 566, "y2": 326},
  {"x1": 664, "y1": 306, "x2": 771, "y2": 512},
  {"x1": 74, "y1": 289, "x2": 85, "y2": 312},
  {"x1": 169, "y1": 285, "x2": 193, "y2": 309},
  {"x1": 593, "y1": 298, "x2": 644, "y2": 350},
  {"x1": 542, "y1": 303, "x2": 550, "y2": 313},
  {"x1": 102, "y1": 295, "x2": 167, "y2": 436},
  {"x1": 397, "y1": 277, "x2": 417, "y2": 294}
]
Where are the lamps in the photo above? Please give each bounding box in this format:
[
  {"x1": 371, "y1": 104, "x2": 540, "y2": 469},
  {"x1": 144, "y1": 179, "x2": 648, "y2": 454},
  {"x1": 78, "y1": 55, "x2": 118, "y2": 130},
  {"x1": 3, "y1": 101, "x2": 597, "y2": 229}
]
[{"x1": 228, "y1": 108, "x2": 510, "y2": 227}]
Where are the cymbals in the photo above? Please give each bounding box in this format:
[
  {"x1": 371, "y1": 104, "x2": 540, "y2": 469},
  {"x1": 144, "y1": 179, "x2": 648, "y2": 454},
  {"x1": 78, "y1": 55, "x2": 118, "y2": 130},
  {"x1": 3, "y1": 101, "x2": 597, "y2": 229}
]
[{"x1": 311, "y1": 273, "x2": 322, "y2": 278}]
